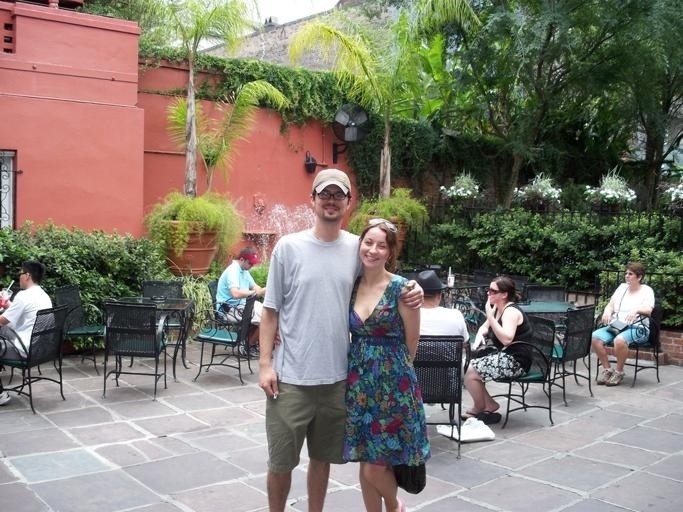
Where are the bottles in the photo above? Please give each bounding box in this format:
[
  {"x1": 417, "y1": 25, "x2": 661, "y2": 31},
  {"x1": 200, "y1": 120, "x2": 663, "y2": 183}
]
[{"x1": 447, "y1": 274, "x2": 454, "y2": 286}]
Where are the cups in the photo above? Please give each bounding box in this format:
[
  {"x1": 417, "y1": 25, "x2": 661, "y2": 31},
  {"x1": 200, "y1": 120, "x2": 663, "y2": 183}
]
[{"x1": 0, "y1": 287, "x2": 13, "y2": 308}]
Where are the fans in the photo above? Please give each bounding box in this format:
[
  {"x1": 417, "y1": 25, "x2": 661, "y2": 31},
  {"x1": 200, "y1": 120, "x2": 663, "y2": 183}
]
[{"x1": 332, "y1": 103, "x2": 369, "y2": 164}]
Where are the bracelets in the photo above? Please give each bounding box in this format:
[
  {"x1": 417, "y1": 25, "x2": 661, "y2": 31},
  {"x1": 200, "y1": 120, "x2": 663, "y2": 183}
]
[{"x1": 249, "y1": 290, "x2": 256, "y2": 296}]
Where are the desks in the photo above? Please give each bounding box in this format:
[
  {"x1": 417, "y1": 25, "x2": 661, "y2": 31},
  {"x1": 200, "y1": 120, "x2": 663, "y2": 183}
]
[{"x1": 519, "y1": 302, "x2": 581, "y2": 388}]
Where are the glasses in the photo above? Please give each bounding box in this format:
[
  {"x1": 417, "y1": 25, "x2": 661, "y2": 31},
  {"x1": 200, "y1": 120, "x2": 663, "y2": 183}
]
[
  {"x1": 318, "y1": 190, "x2": 345, "y2": 200},
  {"x1": 369, "y1": 218, "x2": 398, "y2": 232}
]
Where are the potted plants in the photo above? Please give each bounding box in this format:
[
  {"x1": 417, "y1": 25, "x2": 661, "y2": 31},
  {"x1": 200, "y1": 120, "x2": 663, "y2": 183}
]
[
  {"x1": 348, "y1": 188, "x2": 432, "y2": 260},
  {"x1": 142, "y1": 189, "x2": 248, "y2": 276}
]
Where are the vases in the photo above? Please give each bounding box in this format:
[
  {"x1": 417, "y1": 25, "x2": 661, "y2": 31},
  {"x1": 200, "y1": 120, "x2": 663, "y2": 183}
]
[
  {"x1": 673, "y1": 206, "x2": 683, "y2": 217},
  {"x1": 594, "y1": 202, "x2": 619, "y2": 213},
  {"x1": 449, "y1": 197, "x2": 476, "y2": 209},
  {"x1": 523, "y1": 201, "x2": 550, "y2": 213}
]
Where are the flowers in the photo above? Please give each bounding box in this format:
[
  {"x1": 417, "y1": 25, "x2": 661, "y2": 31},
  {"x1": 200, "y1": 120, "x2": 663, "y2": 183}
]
[
  {"x1": 513, "y1": 169, "x2": 563, "y2": 204},
  {"x1": 439, "y1": 171, "x2": 484, "y2": 200},
  {"x1": 655, "y1": 178, "x2": 683, "y2": 211},
  {"x1": 582, "y1": 166, "x2": 638, "y2": 208}
]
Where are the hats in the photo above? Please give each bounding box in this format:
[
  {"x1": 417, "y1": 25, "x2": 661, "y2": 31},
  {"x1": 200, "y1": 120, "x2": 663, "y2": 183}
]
[
  {"x1": 312, "y1": 169, "x2": 351, "y2": 196},
  {"x1": 417, "y1": 271, "x2": 448, "y2": 291},
  {"x1": 239, "y1": 248, "x2": 259, "y2": 264}
]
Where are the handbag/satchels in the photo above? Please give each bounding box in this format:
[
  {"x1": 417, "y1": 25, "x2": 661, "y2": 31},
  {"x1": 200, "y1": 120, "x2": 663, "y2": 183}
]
[
  {"x1": 607, "y1": 321, "x2": 628, "y2": 335},
  {"x1": 475, "y1": 344, "x2": 498, "y2": 357},
  {"x1": 392, "y1": 463, "x2": 425, "y2": 494}
]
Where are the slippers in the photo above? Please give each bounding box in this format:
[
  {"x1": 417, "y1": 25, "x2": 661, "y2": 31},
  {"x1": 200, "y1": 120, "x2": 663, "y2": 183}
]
[{"x1": 461, "y1": 411, "x2": 501, "y2": 424}]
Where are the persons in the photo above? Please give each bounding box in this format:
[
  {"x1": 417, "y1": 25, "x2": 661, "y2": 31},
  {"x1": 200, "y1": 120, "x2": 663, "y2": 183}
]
[
  {"x1": 216, "y1": 248, "x2": 267, "y2": 358},
  {"x1": 460, "y1": 275, "x2": 533, "y2": 422},
  {"x1": 419, "y1": 270, "x2": 467, "y2": 398},
  {"x1": 0, "y1": 261, "x2": 53, "y2": 404},
  {"x1": 591, "y1": 262, "x2": 655, "y2": 386},
  {"x1": 260, "y1": 169, "x2": 423, "y2": 512},
  {"x1": 347, "y1": 219, "x2": 420, "y2": 509}
]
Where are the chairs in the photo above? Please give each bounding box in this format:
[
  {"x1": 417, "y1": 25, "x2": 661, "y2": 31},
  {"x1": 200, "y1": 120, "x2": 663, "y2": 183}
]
[
  {"x1": 0, "y1": 278, "x2": 256, "y2": 414},
  {"x1": 483, "y1": 312, "x2": 557, "y2": 432},
  {"x1": 599, "y1": 298, "x2": 662, "y2": 389},
  {"x1": 414, "y1": 333, "x2": 464, "y2": 458},
  {"x1": 552, "y1": 302, "x2": 599, "y2": 408}
]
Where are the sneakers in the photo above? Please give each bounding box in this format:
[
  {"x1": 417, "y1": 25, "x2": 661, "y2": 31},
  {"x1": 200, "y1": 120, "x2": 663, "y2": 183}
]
[{"x1": 597, "y1": 369, "x2": 624, "y2": 386}]
